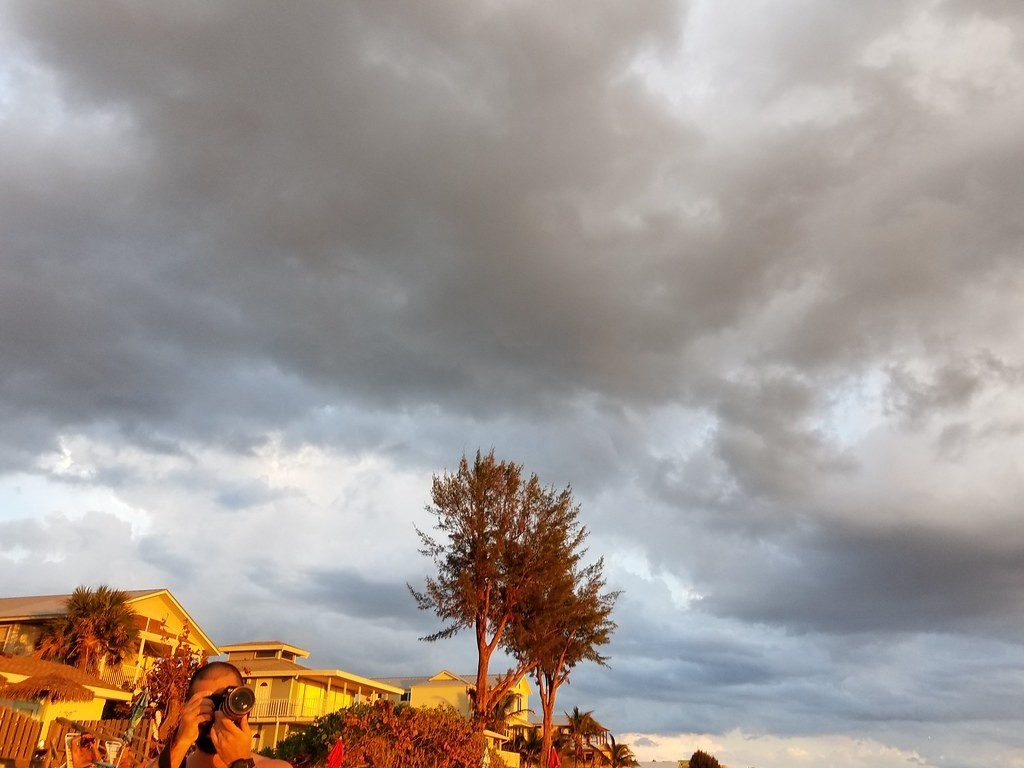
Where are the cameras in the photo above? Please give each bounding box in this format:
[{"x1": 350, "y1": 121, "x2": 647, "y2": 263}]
[{"x1": 198, "y1": 686, "x2": 256, "y2": 728}]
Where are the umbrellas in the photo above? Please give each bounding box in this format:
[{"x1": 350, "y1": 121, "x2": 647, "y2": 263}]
[{"x1": 1, "y1": 670, "x2": 94, "y2": 757}]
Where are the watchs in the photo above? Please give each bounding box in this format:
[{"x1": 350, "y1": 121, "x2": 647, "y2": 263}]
[{"x1": 230, "y1": 758, "x2": 256, "y2": 768}]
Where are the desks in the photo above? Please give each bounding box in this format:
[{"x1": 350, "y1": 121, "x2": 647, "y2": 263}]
[{"x1": 96, "y1": 762, "x2": 114, "y2": 768}]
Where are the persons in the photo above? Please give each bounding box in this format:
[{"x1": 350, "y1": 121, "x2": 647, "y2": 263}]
[
  {"x1": 150, "y1": 662, "x2": 292, "y2": 768},
  {"x1": 72, "y1": 732, "x2": 109, "y2": 768}
]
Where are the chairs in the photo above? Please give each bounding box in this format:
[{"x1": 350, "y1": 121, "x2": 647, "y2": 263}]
[
  {"x1": 60, "y1": 733, "x2": 80, "y2": 768},
  {"x1": 106, "y1": 741, "x2": 123, "y2": 764}
]
